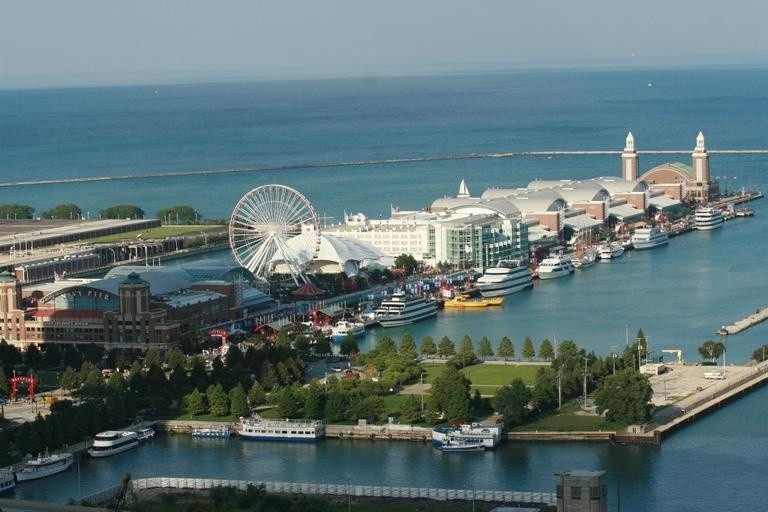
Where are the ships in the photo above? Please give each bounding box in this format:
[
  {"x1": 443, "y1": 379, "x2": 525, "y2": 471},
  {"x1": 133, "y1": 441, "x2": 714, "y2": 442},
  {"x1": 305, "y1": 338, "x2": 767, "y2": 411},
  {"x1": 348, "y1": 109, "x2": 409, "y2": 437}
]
[
  {"x1": 537, "y1": 255, "x2": 575, "y2": 280},
  {"x1": 476, "y1": 259, "x2": 534, "y2": 298},
  {"x1": 631, "y1": 228, "x2": 669, "y2": 250},
  {"x1": 376, "y1": 294, "x2": 438, "y2": 329},
  {"x1": 695, "y1": 207, "x2": 724, "y2": 230}
]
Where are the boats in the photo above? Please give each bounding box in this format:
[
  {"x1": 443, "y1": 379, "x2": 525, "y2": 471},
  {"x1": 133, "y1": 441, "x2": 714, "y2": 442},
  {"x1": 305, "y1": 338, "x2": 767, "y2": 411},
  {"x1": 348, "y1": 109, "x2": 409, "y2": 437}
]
[
  {"x1": 87, "y1": 427, "x2": 156, "y2": 458},
  {"x1": 443, "y1": 294, "x2": 504, "y2": 307},
  {"x1": 601, "y1": 242, "x2": 625, "y2": 259},
  {"x1": 15, "y1": 446, "x2": 74, "y2": 483},
  {"x1": 722, "y1": 209, "x2": 754, "y2": 220},
  {"x1": 331, "y1": 319, "x2": 367, "y2": 342},
  {"x1": 238, "y1": 417, "x2": 326, "y2": 443},
  {"x1": 0, "y1": 469, "x2": 15, "y2": 495},
  {"x1": 431, "y1": 422, "x2": 502, "y2": 453}
]
